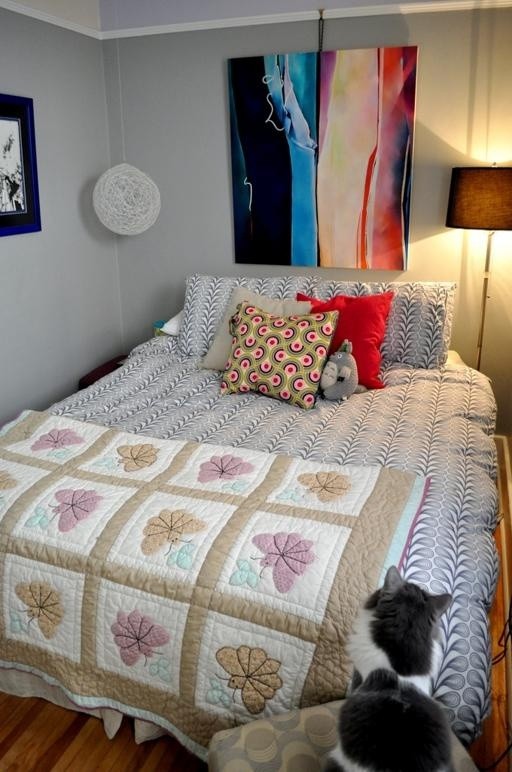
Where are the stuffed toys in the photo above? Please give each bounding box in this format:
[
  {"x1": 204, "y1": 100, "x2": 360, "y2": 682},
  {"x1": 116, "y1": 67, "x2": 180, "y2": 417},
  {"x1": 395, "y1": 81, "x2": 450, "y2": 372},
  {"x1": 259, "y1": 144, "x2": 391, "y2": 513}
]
[{"x1": 319, "y1": 339, "x2": 366, "y2": 402}]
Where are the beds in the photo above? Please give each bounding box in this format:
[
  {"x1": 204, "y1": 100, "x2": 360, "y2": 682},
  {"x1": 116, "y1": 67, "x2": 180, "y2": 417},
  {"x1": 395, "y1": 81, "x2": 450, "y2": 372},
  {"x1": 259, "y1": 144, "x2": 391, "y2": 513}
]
[{"x1": 0, "y1": 322, "x2": 491, "y2": 762}]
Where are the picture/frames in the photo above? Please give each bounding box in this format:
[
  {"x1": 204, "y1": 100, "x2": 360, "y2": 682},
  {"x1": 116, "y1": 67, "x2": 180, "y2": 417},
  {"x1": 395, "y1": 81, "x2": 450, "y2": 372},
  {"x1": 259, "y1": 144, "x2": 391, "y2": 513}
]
[{"x1": 0, "y1": 93, "x2": 42, "y2": 238}]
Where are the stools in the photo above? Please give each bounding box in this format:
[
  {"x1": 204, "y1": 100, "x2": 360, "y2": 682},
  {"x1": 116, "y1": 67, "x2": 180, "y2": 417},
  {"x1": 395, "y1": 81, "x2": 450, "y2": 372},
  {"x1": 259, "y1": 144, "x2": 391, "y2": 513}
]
[{"x1": 205, "y1": 694, "x2": 480, "y2": 772}]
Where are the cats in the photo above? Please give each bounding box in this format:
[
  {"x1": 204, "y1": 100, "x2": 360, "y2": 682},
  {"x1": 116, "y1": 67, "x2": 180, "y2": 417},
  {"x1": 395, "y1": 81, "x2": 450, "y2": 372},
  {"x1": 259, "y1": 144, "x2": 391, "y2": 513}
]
[{"x1": 323, "y1": 565, "x2": 455, "y2": 772}]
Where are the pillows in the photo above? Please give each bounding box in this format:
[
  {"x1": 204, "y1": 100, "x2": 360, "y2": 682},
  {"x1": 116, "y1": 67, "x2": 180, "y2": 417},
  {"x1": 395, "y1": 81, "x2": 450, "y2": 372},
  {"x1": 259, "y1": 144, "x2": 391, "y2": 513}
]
[
  {"x1": 296, "y1": 293, "x2": 393, "y2": 391},
  {"x1": 201, "y1": 287, "x2": 312, "y2": 373},
  {"x1": 221, "y1": 301, "x2": 340, "y2": 410},
  {"x1": 179, "y1": 272, "x2": 322, "y2": 357},
  {"x1": 316, "y1": 279, "x2": 457, "y2": 369}
]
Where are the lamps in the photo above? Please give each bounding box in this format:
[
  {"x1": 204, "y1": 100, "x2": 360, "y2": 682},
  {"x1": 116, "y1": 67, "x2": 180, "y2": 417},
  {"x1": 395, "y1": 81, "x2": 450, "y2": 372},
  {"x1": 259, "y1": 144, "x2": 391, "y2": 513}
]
[{"x1": 446, "y1": 165, "x2": 512, "y2": 371}]
